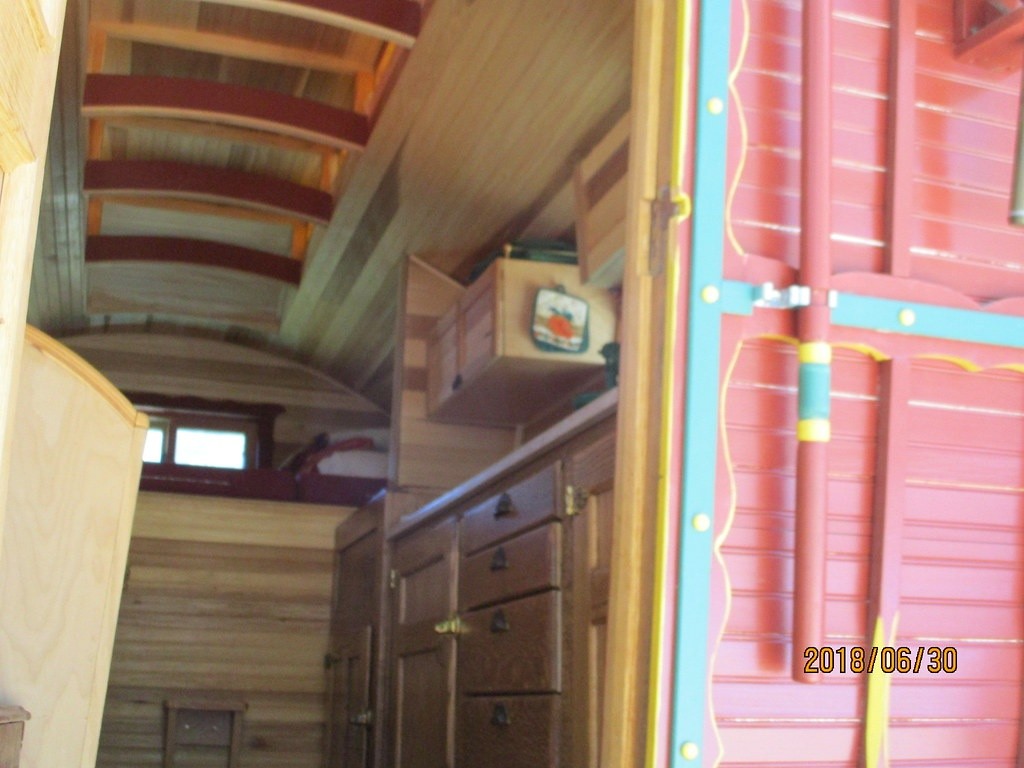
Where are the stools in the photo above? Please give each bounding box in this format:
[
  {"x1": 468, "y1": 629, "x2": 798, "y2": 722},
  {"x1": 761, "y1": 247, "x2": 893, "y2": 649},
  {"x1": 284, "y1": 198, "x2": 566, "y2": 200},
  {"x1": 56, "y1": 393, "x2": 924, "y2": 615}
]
[{"x1": 163, "y1": 698, "x2": 249, "y2": 768}]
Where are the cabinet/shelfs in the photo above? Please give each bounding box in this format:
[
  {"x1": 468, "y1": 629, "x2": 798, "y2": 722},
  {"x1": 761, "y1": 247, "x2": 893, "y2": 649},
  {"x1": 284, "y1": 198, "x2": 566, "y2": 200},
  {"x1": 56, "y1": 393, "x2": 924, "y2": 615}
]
[{"x1": 321, "y1": 108, "x2": 632, "y2": 768}]
